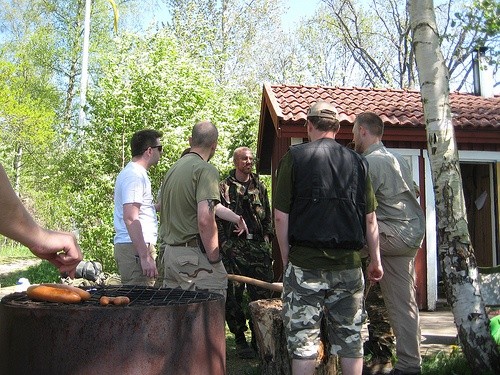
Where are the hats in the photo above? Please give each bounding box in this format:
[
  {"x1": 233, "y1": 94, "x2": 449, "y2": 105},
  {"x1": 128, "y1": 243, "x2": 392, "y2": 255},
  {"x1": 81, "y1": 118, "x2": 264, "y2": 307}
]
[{"x1": 307, "y1": 101, "x2": 340, "y2": 120}]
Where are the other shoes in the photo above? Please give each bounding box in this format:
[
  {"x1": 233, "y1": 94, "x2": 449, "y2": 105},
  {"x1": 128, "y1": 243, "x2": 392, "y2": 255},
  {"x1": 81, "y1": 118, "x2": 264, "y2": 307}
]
[
  {"x1": 236, "y1": 336, "x2": 253, "y2": 358},
  {"x1": 387, "y1": 368, "x2": 422, "y2": 375},
  {"x1": 251, "y1": 331, "x2": 257, "y2": 349}
]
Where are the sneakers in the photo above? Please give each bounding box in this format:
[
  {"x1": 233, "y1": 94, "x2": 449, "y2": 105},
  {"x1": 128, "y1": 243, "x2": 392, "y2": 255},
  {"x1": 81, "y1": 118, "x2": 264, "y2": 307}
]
[{"x1": 362, "y1": 353, "x2": 393, "y2": 375}]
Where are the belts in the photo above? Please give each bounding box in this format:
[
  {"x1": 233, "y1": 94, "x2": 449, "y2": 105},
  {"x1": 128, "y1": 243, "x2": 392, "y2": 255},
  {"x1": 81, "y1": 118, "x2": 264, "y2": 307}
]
[
  {"x1": 240, "y1": 234, "x2": 254, "y2": 240},
  {"x1": 169, "y1": 239, "x2": 200, "y2": 247}
]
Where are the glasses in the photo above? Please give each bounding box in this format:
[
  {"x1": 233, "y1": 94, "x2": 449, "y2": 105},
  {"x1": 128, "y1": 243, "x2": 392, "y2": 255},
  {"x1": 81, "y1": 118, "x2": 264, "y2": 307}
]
[{"x1": 151, "y1": 146, "x2": 162, "y2": 152}]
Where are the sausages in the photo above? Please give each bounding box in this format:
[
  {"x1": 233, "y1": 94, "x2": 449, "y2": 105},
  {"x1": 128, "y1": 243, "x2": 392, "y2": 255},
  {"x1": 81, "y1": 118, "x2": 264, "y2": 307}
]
[
  {"x1": 100, "y1": 296, "x2": 130, "y2": 305},
  {"x1": 27, "y1": 283, "x2": 91, "y2": 303}
]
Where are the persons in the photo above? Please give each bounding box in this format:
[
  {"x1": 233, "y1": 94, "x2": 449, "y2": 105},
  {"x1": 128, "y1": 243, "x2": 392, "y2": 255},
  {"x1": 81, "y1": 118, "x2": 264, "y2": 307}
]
[
  {"x1": 181, "y1": 149, "x2": 249, "y2": 236},
  {"x1": 161, "y1": 121, "x2": 228, "y2": 302},
  {"x1": 0, "y1": 163, "x2": 83, "y2": 281},
  {"x1": 215, "y1": 146, "x2": 274, "y2": 359},
  {"x1": 352, "y1": 112, "x2": 427, "y2": 375},
  {"x1": 347, "y1": 142, "x2": 395, "y2": 375},
  {"x1": 114, "y1": 129, "x2": 162, "y2": 287},
  {"x1": 274, "y1": 100, "x2": 384, "y2": 375}
]
